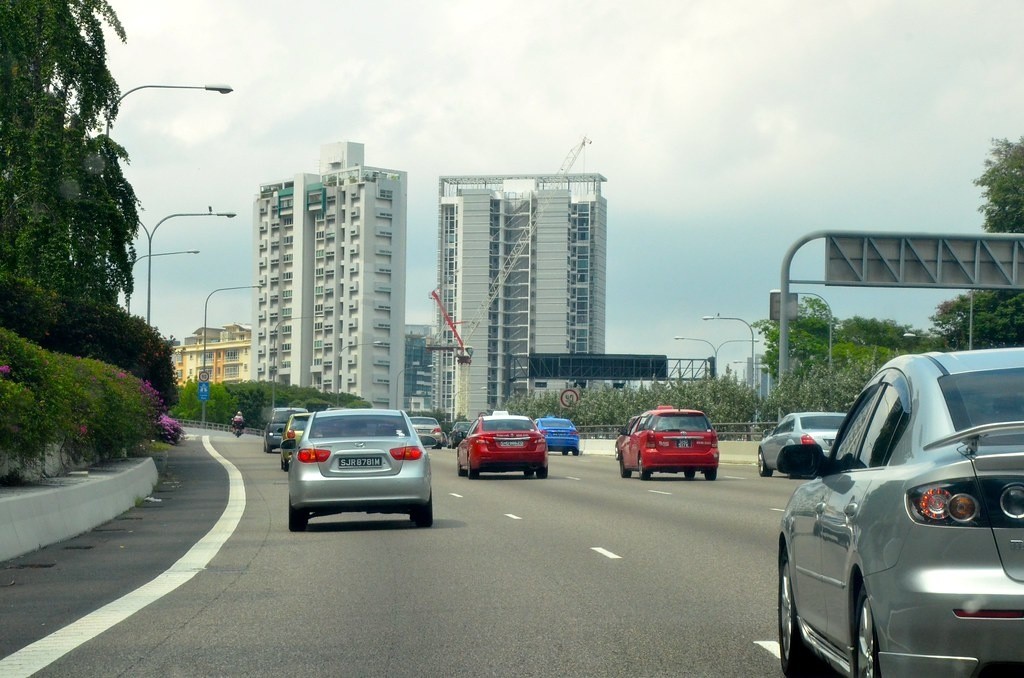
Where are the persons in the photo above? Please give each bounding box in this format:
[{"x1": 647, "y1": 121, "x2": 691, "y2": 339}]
[{"x1": 232, "y1": 410, "x2": 244, "y2": 433}]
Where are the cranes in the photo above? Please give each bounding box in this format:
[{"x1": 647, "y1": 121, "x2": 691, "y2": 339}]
[{"x1": 430, "y1": 133, "x2": 591, "y2": 414}]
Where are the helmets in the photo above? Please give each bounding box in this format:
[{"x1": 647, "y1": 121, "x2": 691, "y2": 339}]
[{"x1": 237, "y1": 412, "x2": 243, "y2": 416}]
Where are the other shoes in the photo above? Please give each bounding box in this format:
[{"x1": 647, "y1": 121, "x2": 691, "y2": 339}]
[
  {"x1": 241, "y1": 432, "x2": 244, "y2": 434},
  {"x1": 233, "y1": 432, "x2": 235, "y2": 434}
]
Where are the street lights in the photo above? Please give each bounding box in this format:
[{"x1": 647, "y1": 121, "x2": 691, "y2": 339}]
[
  {"x1": 100, "y1": 83, "x2": 234, "y2": 349},
  {"x1": 787, "y1": 291, "x2": 834, "y2": 411},
  {"x1": 702, "y1": 315, "x2": 755, "y2": 398},
  {"x1": 201, "y1": 284, "x2": 264, "y2": 425},
  {"x1": 122, "y1": 211, "x2": 240, "y2": 330},
  {"x1": 126, "y1": 250, "x2": 205, "y2": 328},
  {"x1": 675, "y1": 336, "x2": 762, "y2": 381},
  {"x1": 272, "y1": 315, "x2": 326, "y2": 414}
]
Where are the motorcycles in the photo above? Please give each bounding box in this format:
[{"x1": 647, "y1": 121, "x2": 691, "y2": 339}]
[{"x1": 231, "y1": 416, "x2": 244, "y2": 438}]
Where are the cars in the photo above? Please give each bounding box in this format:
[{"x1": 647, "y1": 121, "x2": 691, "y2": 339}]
[
  {"x1": 456, "y1": 413, "x2": 547, "y2": 480},
  {"x1": 614, "y1": 408, "x2": 721, "y2": 480},
  {"x1": 448, "y1": 421, "x2": 475, "y2": 449},
  {"x1": 264, "y1": 405, "x2": 313, "y2": 454},
  {"x1": 288, "y1": 408, "x2": 435, "y2": 532},
  {"x1": 407, "y1": 414, "x2": 444, "y2": 448},
  {"x1": 534, "y1": 415, "x2": 581, "y2": 456},
  {"x1": 282, "y1": 413, "x2": 315, "y2": 471},
  {"x1": 757, "y1": 412, "x2": 850, "y2": 478},
  {"x1": 774, "y1": 345, "x2": 1024, "y2": 678}
]
